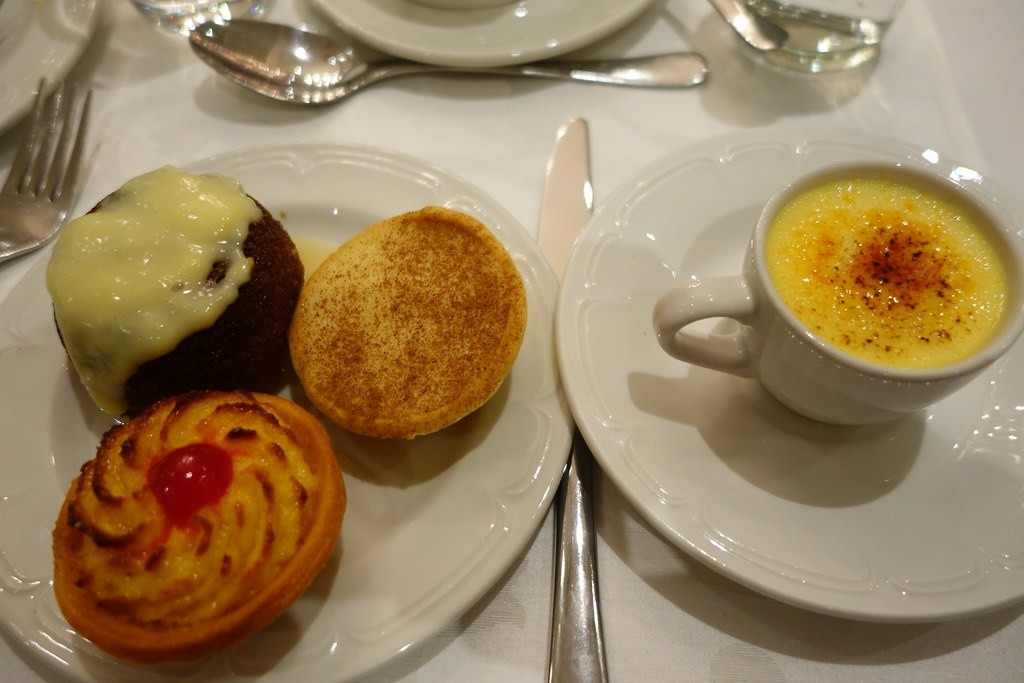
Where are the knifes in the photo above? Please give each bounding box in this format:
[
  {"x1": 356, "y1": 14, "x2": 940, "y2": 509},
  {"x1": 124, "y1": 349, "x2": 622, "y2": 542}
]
[{"x1": 538, "y1": 118, "x2": 610, "y2": 683}]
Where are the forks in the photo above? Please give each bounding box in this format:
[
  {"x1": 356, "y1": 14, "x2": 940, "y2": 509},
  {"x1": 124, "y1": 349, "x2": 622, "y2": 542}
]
[{"x1": 1, "y1": 78, "x2": 93, "y2": 261}]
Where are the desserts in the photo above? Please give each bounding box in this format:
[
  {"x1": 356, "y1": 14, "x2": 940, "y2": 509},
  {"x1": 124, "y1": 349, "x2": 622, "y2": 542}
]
[
  {"x1": 44, "y1": 165, "x2": 304, "y2": 417},
  {"x1": 54, "y1": 390, "x2": 347, "y2": 663},
  {"x1": 290, "y1": 206, "x2": 528, "y2": 439}
]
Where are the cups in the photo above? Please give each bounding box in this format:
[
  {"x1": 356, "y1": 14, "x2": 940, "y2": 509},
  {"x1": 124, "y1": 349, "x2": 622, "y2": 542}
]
[
  {"x1": 133, "y1": 1, "x2": 276, "y2": 39},
  {"x1": 651, "y1": 158, "x2": 1024, "y2": 423},
  {"x1": 742, "y1": 1, "x2": 907, "y2": 77}
]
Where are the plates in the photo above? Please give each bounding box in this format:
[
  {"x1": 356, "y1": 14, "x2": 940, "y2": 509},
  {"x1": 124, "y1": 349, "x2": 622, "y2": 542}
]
[
  {"x1": 309, "y1": 1, "x2": 656, "y2": 68},
  {"x1": 554, "y1": 126, "x2": 1024, "y2": 621},
  {"x1": 0, "y1": 1, "x2": 100, "y2": 136},
  {"x1": 0, "y1": 146, "x2": 574, "y2": 682}
]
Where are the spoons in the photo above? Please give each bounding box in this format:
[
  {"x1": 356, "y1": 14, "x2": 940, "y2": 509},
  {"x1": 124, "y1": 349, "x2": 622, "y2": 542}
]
[{"x1": 190, "y1": 19, "x2": 708, "y2": 104}]
